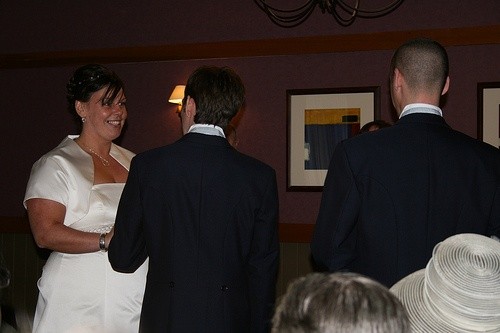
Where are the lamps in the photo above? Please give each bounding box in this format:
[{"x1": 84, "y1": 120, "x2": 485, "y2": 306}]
[
  {"x1": 255, "y1": 0, "x2": 404, "y2": 28},
  {"x1": 168, "y1": 84, "x2": 186, "y2": 118}
]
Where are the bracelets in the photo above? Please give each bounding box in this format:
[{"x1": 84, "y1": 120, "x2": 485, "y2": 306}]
[{"x1": 98, "y1": 233, "x2": 107, "y2": 252}]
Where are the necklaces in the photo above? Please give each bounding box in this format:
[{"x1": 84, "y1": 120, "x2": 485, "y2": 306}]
[{"x1": 85, "y1": 145, "x2": 110, "y2": 166}]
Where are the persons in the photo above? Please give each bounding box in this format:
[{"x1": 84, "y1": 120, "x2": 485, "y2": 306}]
[
  {"x1": 309, "y1": 37, "x2": 500, "y2": 287},
  {"x1": 360, "y1": 119, "x2": 392, "y2": 136},
  {"x1": 107, "y1": 67, "x2": 280, "y2": 333},
  {"x1": 22, "y1": 63, "x2": 148, "y2": 333},
  {"x1": 271, "y1": 271, "x2": 413, "y2": 333}
]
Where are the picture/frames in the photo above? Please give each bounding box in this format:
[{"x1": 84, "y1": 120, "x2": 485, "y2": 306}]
[
  {"x1": 286, "y1": 86, "x2": 381, "y2": 192},
  {"x1": 477, "y1": 82, "x2": 500, "y2": 150}
]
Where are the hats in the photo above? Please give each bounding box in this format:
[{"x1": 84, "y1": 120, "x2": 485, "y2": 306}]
[{"x1": 388, "y1": 232, "x2": 500, "y2": 333}]
